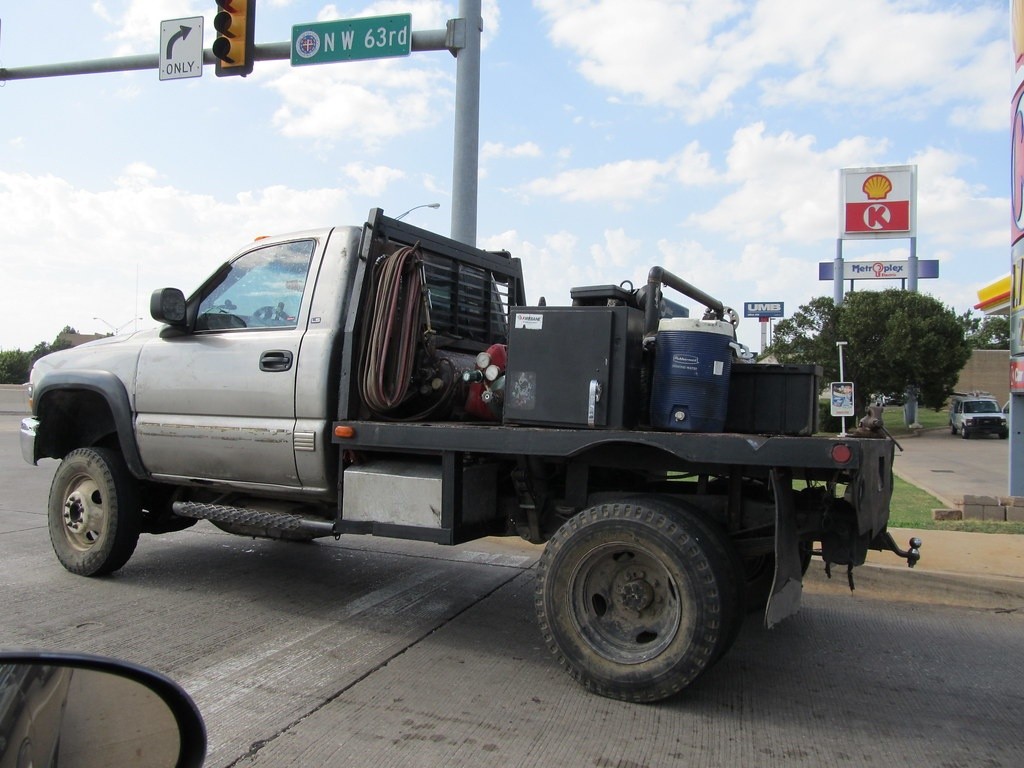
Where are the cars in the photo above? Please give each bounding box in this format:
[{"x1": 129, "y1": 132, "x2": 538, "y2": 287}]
[{"x1": 874, "y1": 392, "x2": 906, "y2": 407}]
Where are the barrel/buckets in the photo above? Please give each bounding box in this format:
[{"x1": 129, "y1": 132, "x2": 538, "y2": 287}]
[{"x1": 650, "y1": 317, "x2": 750, "y2": 434}]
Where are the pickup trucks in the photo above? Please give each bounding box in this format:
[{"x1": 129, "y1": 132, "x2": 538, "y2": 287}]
[{"x1": 18, "y1": 207, "x2": 923, "y2": 706}]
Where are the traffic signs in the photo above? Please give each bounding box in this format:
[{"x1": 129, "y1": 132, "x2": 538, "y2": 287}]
[{"x1": 159, "y1": 15, "x2": 205, "y2": 79}]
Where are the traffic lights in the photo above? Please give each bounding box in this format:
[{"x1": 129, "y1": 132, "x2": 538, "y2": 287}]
[{"x1": 212, "y1": 0, "x2": 255, "y2": 77}]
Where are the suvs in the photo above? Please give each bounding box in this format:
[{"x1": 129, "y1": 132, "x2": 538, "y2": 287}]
[{"x1": 949, "y1": 390, "x2": 1010, "y2": 439}]
